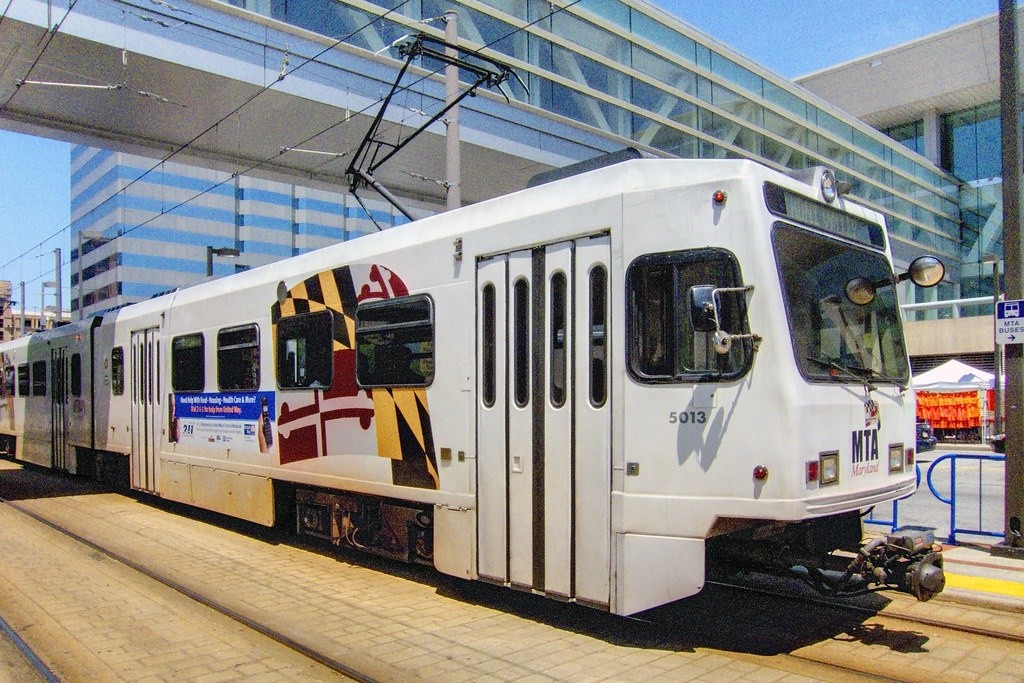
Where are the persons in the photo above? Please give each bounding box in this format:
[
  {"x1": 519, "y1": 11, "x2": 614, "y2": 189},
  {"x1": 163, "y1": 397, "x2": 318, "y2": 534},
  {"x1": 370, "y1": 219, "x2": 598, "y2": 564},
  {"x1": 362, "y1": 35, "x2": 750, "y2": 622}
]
[{"x1": 377, "y1": 346, "x2": 426, "y2": 385}]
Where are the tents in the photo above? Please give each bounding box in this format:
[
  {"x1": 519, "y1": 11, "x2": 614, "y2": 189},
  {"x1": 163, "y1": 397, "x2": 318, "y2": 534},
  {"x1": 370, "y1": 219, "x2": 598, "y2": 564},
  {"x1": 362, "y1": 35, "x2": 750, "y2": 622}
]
[{"x1": 912, "y1": 358, "x2": 1006, "y2": 445}]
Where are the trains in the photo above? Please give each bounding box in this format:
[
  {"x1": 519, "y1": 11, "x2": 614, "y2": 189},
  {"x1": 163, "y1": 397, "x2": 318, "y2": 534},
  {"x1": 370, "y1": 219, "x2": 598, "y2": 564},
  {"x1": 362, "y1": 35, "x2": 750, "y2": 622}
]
[{"x1": 0, "y1": 146, "x2": 944, "y2": 617}]
